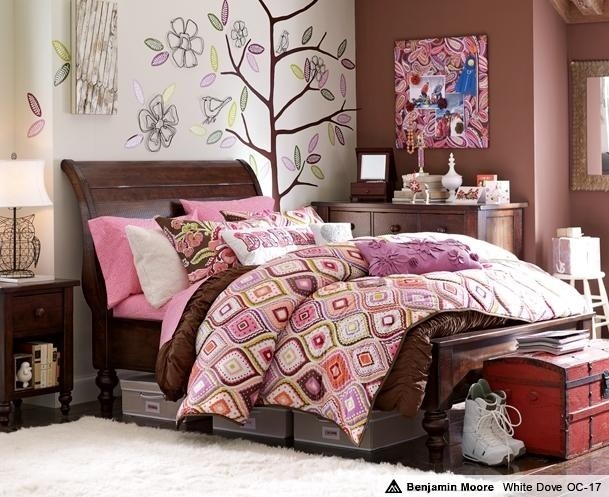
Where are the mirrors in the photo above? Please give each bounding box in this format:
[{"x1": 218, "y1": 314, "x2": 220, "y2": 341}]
[
  {"x1": 570, "y1": 59, "x2": 608, "y2": 192},
  {"x1": 350, "y1": 148, "x2": 398, "y2": 203}
]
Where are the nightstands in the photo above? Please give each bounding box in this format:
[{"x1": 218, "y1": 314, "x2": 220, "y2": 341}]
[{"x1": 0, "y1": 278, "x2": 81, "y2": 432}]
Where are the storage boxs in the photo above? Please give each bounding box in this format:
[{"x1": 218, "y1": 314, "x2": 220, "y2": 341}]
[
  {"x1": 481, "y1": 338, "x2": 608, "y2": 462},
  {"x1": 551, "y1": 237, "x2": 601, "y2": 276}
]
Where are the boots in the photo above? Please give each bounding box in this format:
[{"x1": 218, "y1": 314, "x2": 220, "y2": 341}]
[{"x1": 460, "y1": 375, "x2": 526, "y2": 466}]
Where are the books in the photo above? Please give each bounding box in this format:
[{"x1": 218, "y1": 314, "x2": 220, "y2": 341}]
[
  {"x1": 516, "y1": 324, "x2": 591, "y2": 353},
  {"x1": 391, "y1": 172, "x2": 449, "y2": 202},
  {"x1": 24, "y1": 341, "x2": 60, "y2": 391},
  {"x1": 476, "y1": 174, "x2": 497, "y2": 187}
]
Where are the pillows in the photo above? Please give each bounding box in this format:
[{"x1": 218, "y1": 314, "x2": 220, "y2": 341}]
[
  {"x1": 354, "y1": 237, "x2": 483, "y2": 277},
  {"x1": 87, "y1": 195, "x2": 353, "y2": 310}
]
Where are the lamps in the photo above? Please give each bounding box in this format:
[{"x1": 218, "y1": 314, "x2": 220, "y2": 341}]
[{"x1": 0, "y1": 158, "x2": 53, "y2": 279}]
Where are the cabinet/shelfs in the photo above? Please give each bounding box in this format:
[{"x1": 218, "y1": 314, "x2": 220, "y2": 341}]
[{"x1": 313, "y1": 200, "x2": 528, "y2": 260}]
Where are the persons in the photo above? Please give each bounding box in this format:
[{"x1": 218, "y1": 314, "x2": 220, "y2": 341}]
[
  {"x1": 421, "y1": 82, "x2": 430, "y2": 98},
  {"x1": 433, "y1": 82, "x2": 444, "y2": 99}
]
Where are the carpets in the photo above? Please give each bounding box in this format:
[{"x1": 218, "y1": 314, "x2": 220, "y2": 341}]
[{"x1": 1, "y1": 415, "x2": 523, "y2": 497}]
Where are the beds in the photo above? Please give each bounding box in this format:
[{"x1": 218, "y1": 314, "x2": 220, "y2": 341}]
[{"x1": 59, "y1": 159, "x2": 598, "y2": 475}]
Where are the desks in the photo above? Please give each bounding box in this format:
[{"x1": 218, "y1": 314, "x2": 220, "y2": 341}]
[{"x1": 550, "y1": 273, "x2": 608, "y2": 340}]
